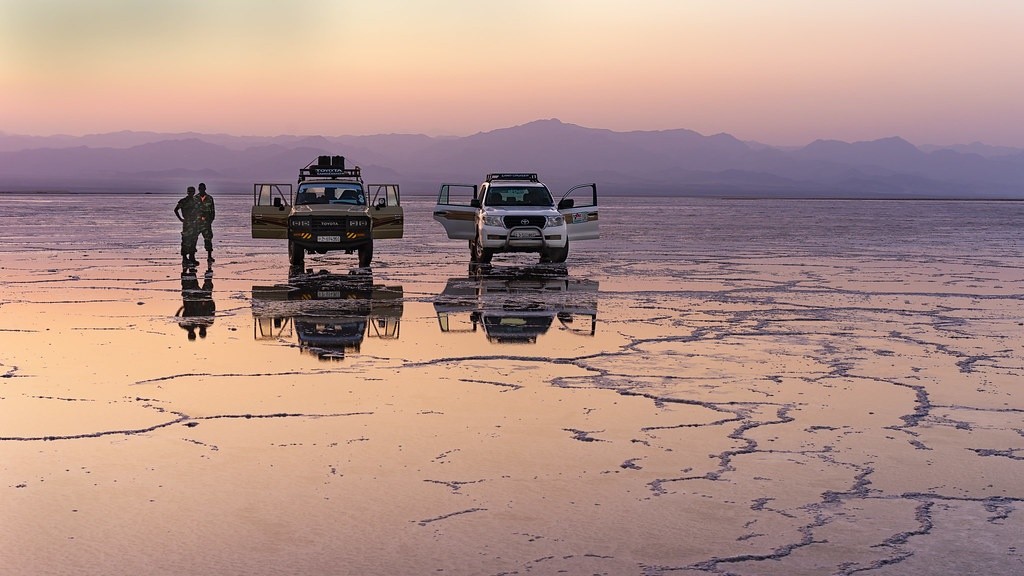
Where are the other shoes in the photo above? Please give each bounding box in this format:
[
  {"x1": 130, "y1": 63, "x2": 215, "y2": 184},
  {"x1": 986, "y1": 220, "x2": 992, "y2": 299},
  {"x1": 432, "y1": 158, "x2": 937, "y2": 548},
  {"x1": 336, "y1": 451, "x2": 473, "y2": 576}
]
[
  {"x1": 189, "y1": 256, "x2": 199, "y2": 264},
  {"x1": 182, "y1": 260, "x2": 196, "y2": 267},
  {"x1": 208, "y1": 257, "x2": 215, "y2": 261}
]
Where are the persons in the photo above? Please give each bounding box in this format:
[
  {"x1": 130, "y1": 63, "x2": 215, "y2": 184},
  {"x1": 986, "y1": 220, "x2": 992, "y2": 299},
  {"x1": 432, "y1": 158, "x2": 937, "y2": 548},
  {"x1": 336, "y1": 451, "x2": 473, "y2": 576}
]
[
  {"x1": 176, "y1": 267, "x2": 203, "y2": 341},
  {"x1": 197, "y1": 265, "x2": 216, "y2": 338},
  {"x1": 174, "y1": 186, "x2": 202, "y2": 267},
  {"x1": 194, "y1": 182, "x2": 215, "y2": 274}
]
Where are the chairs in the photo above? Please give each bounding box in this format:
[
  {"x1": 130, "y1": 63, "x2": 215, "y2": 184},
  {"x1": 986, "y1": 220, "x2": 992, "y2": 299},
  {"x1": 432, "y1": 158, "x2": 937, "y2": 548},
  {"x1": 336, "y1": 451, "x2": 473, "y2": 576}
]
[
  {"x1": 488, "y1": 193, "x2": 505, "y2": 205},
  {"x1": 338, "y1": 189, "x2": 359, "y2": 203},
  {"x1": 301, "y1": 192, "x2": 317, "y2": 203}
]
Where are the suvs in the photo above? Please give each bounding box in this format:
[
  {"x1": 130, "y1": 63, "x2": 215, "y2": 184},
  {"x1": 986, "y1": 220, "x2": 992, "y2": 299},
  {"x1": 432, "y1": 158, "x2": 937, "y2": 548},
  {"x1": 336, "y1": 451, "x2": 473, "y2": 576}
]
[
  {"x1": 251, "y1": 178, "x2": 404, "y2": 266},
  {"x1": 252, "y1": 264, "x2": 404, "y2": 362},
  {"x1": 434, "y1": 262, "x2": 599, "y2": 344},
  {"x1": 433, "y1": 172, "x2": 599, "y2": 264}
]
